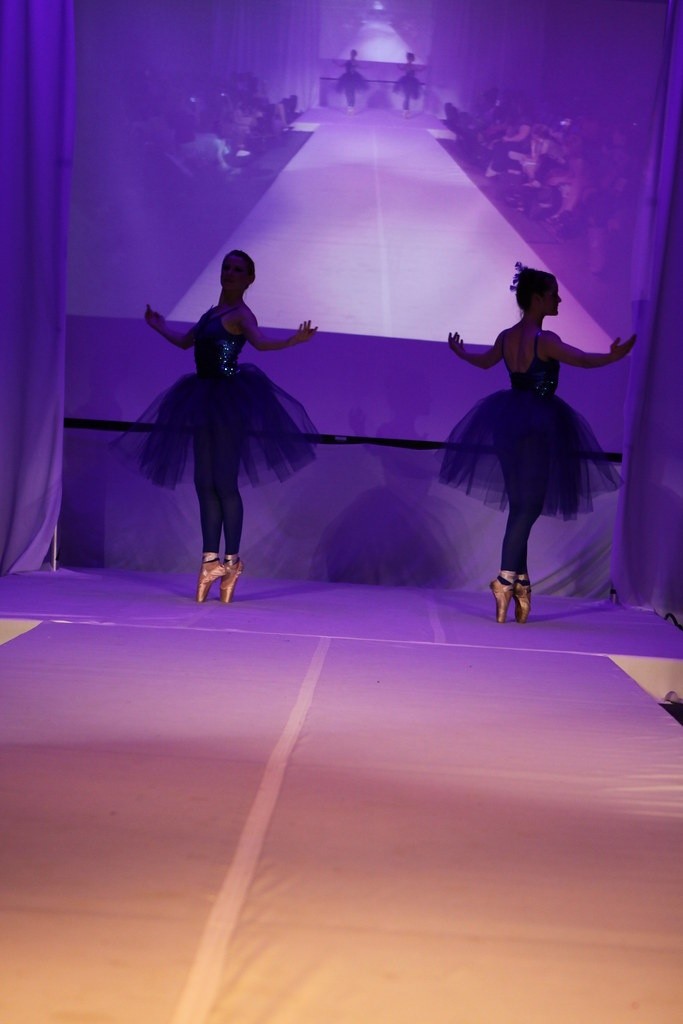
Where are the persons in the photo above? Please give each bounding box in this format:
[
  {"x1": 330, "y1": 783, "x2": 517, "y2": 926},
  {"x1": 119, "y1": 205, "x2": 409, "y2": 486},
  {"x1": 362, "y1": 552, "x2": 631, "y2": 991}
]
[
  {"x1": 329, "y1": 51, "x2": 369, "y2": 117},
  {"x1": 463, "y1": 90, "x2": 647, "y2": 276},
  {"x1": 132, "y1": 75, "x2": 296, "y2": 195},
  {"x1": 108, "y1": 250, "x2": 318, "y2": 602},
  {"x1": 393, "y1": 53, "x2": 427, "y2": 118},
  {"x1": 441, "y1": 264, "x2": 636, "y2": 623}
]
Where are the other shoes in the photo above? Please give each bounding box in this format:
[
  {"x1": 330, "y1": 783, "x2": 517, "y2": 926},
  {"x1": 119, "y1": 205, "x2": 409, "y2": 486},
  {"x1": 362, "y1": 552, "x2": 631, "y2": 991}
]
[
  {"x1": 514, "y1": 582, "x2": 530, "y2": 622},
  {"x1": 195, "y1": 564, "x2": 226, "y2": 603},
  {"x1": 490, "y1": 579, "x2": 514, "y2": 623},
  {"x1": 218, "y1": 561, "x2": 244, "y2": 604}
]
